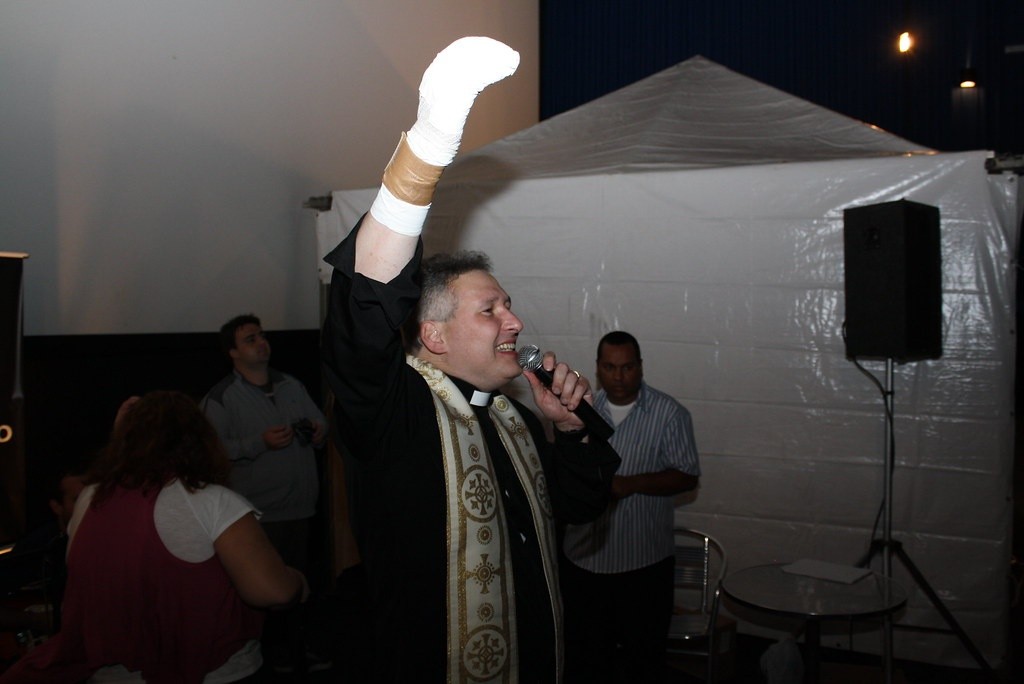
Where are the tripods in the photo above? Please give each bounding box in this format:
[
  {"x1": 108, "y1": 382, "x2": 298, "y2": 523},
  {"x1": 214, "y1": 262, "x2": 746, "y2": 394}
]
[{"x1": 854, "y1": 357, "x2": 1001, "y2": 684}]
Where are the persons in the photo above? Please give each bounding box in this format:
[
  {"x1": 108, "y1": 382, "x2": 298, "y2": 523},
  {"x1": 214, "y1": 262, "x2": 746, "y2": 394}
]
[
  {"x1": 559, "y1": 331, "x2": 702, "y2": 684},
  {"x1": 311, "y1": 37, "x2": 624, "y2": 684},
  {"x1": 1, "y1": 391, "x2": 304, "y2": 684},
  {"x1": 200, "y1": 316, "x2": 332, "y2": 673}
]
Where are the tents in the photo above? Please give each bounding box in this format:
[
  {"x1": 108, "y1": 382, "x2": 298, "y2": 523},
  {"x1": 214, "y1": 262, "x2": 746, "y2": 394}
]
[{"x1": 316, "y1": 52, "x2": 1024, "y2": 669}]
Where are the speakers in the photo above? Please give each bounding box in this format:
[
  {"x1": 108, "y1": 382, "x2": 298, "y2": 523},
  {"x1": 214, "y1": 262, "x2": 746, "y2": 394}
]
[{"x1": 839, "y1": 199, "x2": 943, "y2": 364}]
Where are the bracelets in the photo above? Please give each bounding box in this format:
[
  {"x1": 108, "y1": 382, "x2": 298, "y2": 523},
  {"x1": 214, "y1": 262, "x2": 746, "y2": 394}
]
[{"x1": 14, "y1": 626, "x2": 29, "y2": 646}]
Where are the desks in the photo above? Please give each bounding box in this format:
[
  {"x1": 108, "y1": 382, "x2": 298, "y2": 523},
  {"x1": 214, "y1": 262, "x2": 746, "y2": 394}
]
[{"x1": 722, "y1": 564, "x2": 908, "y2": 684}]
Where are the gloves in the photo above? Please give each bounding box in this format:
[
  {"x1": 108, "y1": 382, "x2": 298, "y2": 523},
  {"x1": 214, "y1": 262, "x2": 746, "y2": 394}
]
[{"x1": 370, "y1": 35, "x2": 521, "y2": 237}]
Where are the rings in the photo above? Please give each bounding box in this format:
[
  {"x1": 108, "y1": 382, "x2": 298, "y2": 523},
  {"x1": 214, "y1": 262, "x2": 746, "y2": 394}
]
[{"x1": 574, "y1": 371, "x2": 580, "y2": 378}]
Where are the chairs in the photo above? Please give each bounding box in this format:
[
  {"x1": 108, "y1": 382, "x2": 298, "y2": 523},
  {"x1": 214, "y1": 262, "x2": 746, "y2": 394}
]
[{"x1": 666, "y1": 526, "x2": 729, "y2": 684}]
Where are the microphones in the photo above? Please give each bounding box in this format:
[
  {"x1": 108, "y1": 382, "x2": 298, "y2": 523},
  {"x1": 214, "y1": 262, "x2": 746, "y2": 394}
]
[{"x1": 515, "y1": 344, "x2": 615, "y2": 442}]
